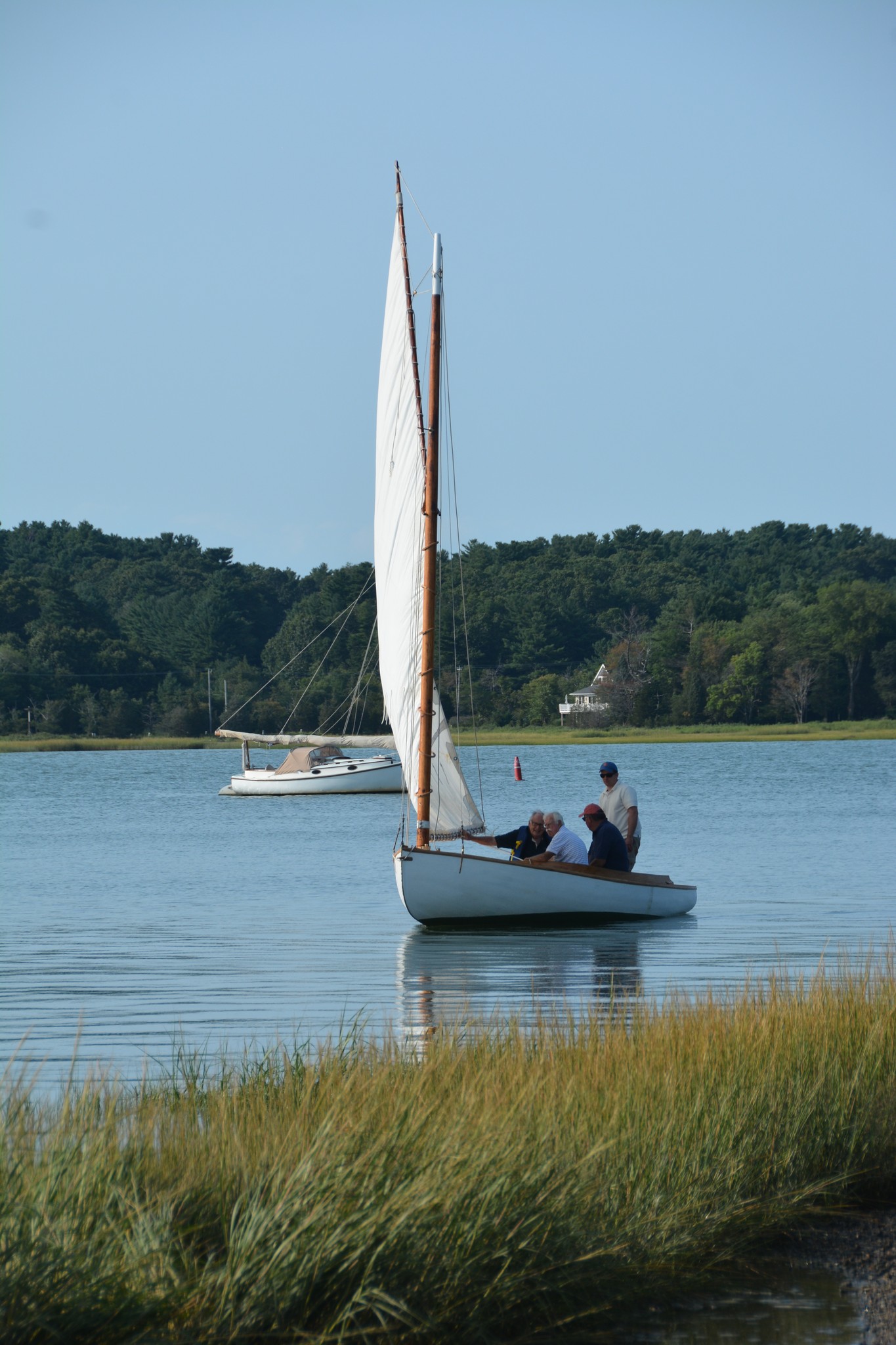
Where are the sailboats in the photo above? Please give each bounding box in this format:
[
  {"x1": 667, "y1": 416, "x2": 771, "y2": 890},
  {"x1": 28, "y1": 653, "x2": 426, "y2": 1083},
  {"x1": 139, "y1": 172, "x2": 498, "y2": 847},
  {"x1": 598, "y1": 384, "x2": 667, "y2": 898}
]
[
  {"x1": 372, "y1": 156, "x2": 702, "y2": 939},
  {"x1": 215, "y1": 565, "x2": 407, "y2": 798}
]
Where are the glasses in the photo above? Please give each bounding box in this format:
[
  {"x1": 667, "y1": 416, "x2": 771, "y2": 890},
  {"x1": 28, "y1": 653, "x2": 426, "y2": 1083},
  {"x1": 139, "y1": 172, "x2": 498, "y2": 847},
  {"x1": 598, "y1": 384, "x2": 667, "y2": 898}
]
[
  {"x1": 531, "y1": 820, "x2": 544, "y2": 828},
  {"x1": 544, "y1": 821, "x2": 557, "y2": 828},
  {"x1": 600, "y1": 773, "x2": 614, "y2": 778},
  {"x1": 582, "y1": 817, "x2": 589, "y2": 821}
]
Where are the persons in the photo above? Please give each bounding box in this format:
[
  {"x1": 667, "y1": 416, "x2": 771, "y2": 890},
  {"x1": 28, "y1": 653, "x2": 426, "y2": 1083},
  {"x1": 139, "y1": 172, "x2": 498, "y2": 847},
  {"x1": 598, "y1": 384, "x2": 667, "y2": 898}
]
[
  {"x1": 524, "y1": 812, "x2": 589, "y2": 865},
  {"x1": 579, "y1": 803, "x2": 629, "y2": 872},
  {"x1": 598, "y1": 761, "x2": 642, "y2": 872},
  {"x1": 460, "y1": 811, "x2": 553, "y2": 861}
]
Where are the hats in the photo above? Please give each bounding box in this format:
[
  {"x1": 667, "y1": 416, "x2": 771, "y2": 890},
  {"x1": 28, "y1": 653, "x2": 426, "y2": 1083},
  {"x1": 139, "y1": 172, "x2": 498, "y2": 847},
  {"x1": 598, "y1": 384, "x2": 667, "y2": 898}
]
[
  {"x1": 579, "y1": 803, "x2": 604, "y2": 818},
  {"x1": 599, "y1": 761, "x2": 618, "y2": 773}
]
